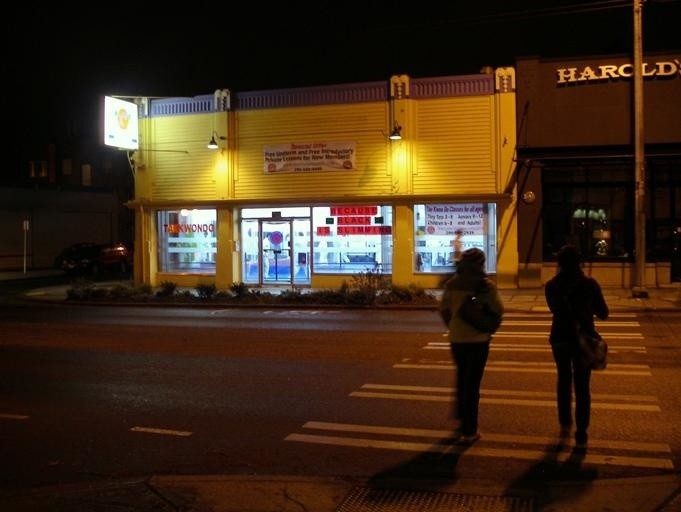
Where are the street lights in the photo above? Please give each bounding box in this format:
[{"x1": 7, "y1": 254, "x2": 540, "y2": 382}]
[{"x1": 22, "y1": 215, "x2": 30, "y2": 276}]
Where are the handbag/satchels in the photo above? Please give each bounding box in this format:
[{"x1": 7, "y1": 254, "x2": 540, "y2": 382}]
[
  {"x1": 456, "y1": 293, "x2": 503, "y2": 334},
  {"x1": 569, "y1": 317, "x2": 609, "y2": 371}
]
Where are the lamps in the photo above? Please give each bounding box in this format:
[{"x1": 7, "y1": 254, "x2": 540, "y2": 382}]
[
  {"x1": 388, "y1": 121, "x2": 403, "y2": 140},
  {"x1": 207, "y1": 129, "x2": 226, "y2": 149}
]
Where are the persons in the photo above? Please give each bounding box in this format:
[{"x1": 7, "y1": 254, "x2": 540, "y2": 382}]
[
  {"x1": 262, "y1": 232, "x2": 272, "y2": 278},
  {"x1": 439, "y1": 248, "x2": 503, "y2": 444},
  {"x1": 545, "y1": 244, "x2": 610, "y2": 443}
]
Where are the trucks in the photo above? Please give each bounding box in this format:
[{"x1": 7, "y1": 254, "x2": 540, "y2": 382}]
[{"x1": 64, "y1": 240, "x2": 129, "y2": 279}]
